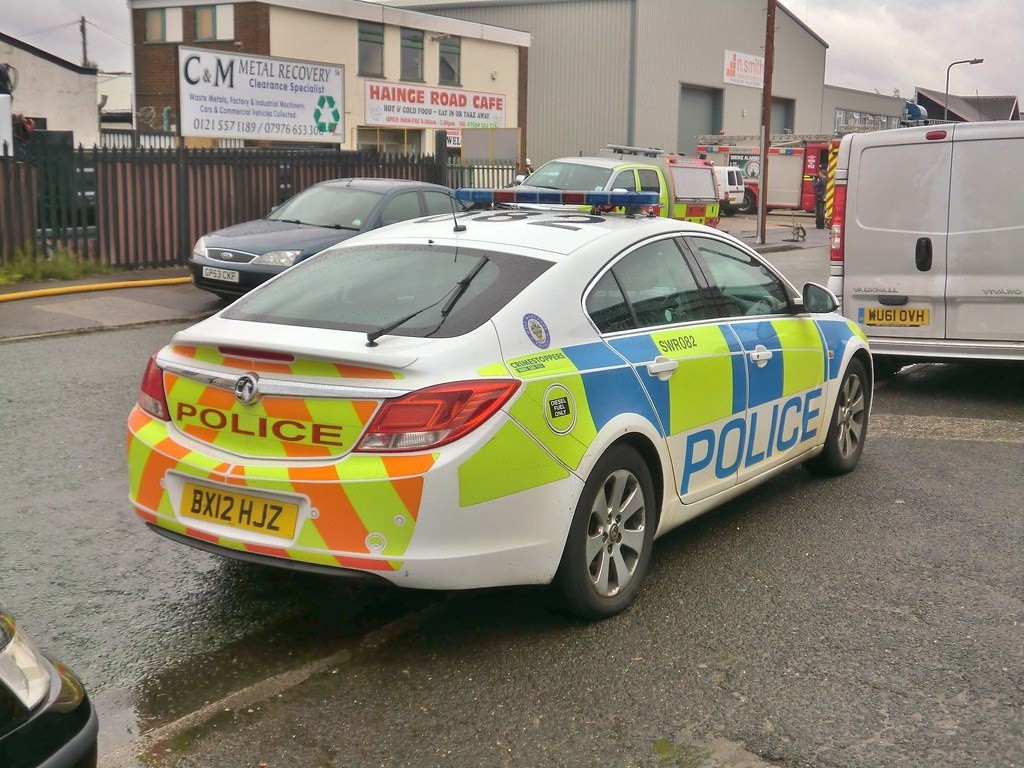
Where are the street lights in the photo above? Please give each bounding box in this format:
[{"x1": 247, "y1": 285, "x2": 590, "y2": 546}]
[{"x1": 944, "y1": 58, "x2": 984, "y2": 119}]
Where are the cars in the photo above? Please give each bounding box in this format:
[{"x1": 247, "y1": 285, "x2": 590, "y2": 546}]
[
  {"x1": 124, "y1": 187, "x2": 877, "y2": 620},
  {"x1": 188, "y1": 180, "x2": 491, "y2": 300}
]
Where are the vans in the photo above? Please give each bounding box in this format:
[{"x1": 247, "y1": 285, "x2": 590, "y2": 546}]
[
  {"x1": 713, "y1": 165, "x2": 746, "y2": 215},
  {"x1": 829, "y1": 121, "x2": 1023, "y2": 378}
]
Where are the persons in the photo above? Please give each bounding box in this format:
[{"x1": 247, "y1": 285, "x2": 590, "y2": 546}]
[
  {"x1": 526, "y1": 157, "x2": 534, "y2": 176},
  {"x1": 812, "y1": 169, "x2": 829, "y2": 229}
]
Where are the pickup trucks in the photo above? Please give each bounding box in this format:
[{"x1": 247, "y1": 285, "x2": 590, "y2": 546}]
[{"x1": 489, "y1": 144, "x2": 722, "y2": 243}]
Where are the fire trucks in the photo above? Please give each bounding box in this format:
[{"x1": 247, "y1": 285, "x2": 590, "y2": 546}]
[{"x1": 694, "y1": 139, "x2": 840, "y2": 213}]
